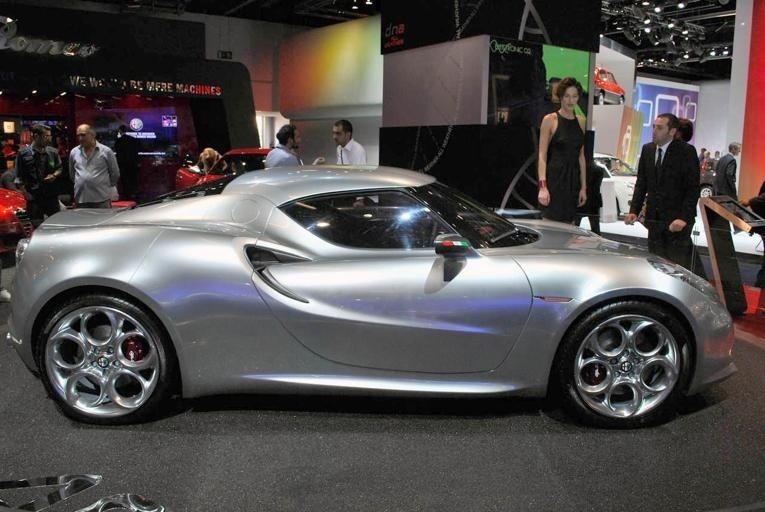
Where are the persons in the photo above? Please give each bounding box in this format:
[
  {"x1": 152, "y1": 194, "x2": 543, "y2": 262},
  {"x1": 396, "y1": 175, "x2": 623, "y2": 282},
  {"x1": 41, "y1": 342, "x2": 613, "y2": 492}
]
[
  {"x1": 575, "y1": 158, "x2": 603, "y2": 235},
  {"x1": 538, "y1": 77, "x2": 587, "y2": 224},
  {"x1": 200, "y1": 147, "x2": 228, "y2": 176},
  {"x1": 265, "y1": 125, "x2": 326, "y2": 168},
  {"x1": 69, "y1": 124, "x2": 120, "y2": 208},
  {"x1": 625, "y1": 113, "x2": 741, "y2": 281},
  {"x1": 332, "y1": 120, "x2": 380, "y2": 209},
  {"x1": 15, "y1": 124, "x2": 64, "y2": 228},
  {"x1": 114, "y1": 125, "x2": 138, "y2": 194}
]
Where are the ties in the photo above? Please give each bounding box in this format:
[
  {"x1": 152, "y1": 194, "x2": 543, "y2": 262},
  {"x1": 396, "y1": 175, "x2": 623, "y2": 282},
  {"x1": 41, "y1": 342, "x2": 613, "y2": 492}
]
[
  {"x1": 655, "y1": 148, "x2": 662, "y2": 184},
  {"x1": 341, "y1": 149, "x2": 343, "y2": 164}
]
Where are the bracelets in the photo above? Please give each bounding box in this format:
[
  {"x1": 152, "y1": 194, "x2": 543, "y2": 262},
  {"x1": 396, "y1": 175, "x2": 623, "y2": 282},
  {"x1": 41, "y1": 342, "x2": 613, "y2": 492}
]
[{"x1": 539, "y1": 180, "x2": 547, "y2": 189}]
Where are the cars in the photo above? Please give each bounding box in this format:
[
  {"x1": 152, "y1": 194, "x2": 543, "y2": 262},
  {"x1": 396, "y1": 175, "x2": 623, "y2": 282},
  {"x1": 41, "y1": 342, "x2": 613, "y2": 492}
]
[
  {"x1": 699, "y1": 166, "x2": 718, "y2": 200},
  {"x1": 594, "y1": 151, "x2": 649, "y2": 215},
  {"x1": 0, "y1": 186, "x2": 32, "y2": 255},
  {"x1": 176, "y1": 148, "x2": 277, "y2": 190}
]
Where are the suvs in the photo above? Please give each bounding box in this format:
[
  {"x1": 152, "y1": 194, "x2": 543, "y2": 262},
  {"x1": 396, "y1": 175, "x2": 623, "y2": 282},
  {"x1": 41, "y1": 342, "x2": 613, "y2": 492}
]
[{"x1": 594, "y1": 66, "x2": 626, "y2": 106}]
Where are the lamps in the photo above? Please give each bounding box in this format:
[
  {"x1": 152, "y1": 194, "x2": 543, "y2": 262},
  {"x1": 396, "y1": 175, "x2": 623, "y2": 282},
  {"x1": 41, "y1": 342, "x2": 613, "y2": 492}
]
[
  {"x1": 352, "y1": 0, "x2": 373, "y2": 10},
  {"x1": 599, "y1": 1, "x2": 731, "y2": 67}
]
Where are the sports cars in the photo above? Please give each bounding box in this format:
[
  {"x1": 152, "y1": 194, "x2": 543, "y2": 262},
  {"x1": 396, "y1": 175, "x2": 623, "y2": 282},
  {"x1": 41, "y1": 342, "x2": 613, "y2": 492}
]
[{"x1": 9, "y1": 164, "x2": 737, "y2": 427}]
